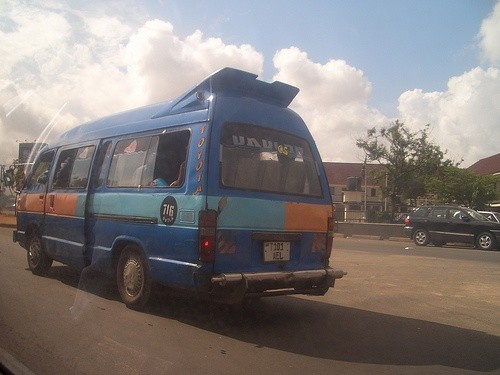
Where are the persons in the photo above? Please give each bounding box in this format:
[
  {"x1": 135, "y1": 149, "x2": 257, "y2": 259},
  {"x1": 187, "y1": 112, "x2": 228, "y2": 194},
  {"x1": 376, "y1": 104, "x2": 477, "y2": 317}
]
[
  {"x1": 16, "y1": 142, "x2": 309, "y2": 203},
  {"x1": 471, "y1": 205, "x2": 481, "y2": 249},
  {"x1": 0, "y1": 177, "x2": 6, "y2": 198}
]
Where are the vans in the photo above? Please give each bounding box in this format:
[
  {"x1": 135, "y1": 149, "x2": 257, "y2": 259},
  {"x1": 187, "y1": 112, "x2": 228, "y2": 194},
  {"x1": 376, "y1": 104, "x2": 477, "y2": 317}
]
[{"x1": 1, "y1": 65, "x2": 348, "y2": 311}]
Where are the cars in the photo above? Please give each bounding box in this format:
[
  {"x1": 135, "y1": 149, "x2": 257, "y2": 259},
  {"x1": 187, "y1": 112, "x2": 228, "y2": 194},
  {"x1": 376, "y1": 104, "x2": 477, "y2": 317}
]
[{"x1": 477, "y1": 210, "x2": 500, "y2": 228}]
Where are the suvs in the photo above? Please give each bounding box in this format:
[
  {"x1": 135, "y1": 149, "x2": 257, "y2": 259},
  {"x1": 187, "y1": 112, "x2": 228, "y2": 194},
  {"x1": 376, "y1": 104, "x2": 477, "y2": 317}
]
[{"x1": 403, "y1": 202, "x2": 500, "y2": 251}]
